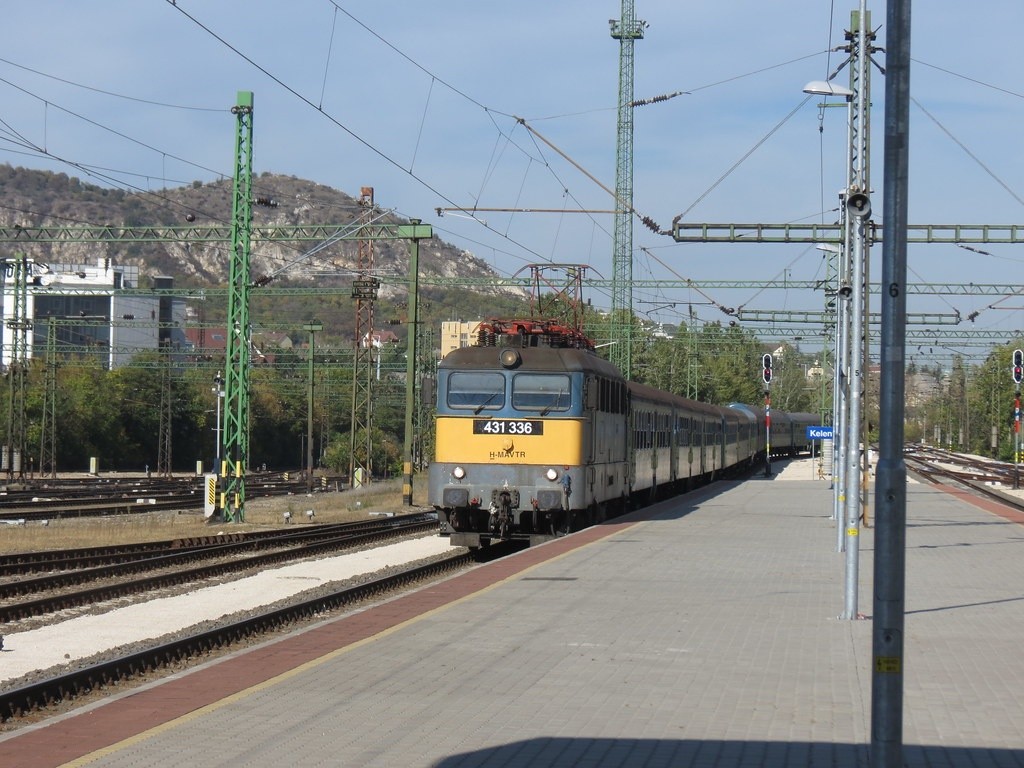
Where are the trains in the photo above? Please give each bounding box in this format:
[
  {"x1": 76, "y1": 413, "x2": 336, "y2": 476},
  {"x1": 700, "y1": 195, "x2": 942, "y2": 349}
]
[{"x1": 420, "y1": 316, "x2": 821, "y2": 549}]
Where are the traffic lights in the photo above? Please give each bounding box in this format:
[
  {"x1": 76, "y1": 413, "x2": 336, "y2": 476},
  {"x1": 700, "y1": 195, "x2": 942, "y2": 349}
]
[
  {"x1": 764, "y1": 389, "x2": 770, "y2": 396},
  {"x1": 763, "y1": 353, "x2": 772, "y2": 384},
  {"x1": 1013, "y1": 349, "x2": 1024, "y2": 385},
  {"x1": 1014, "y1": 389, "x2": 1022, "y2": 399}
]
